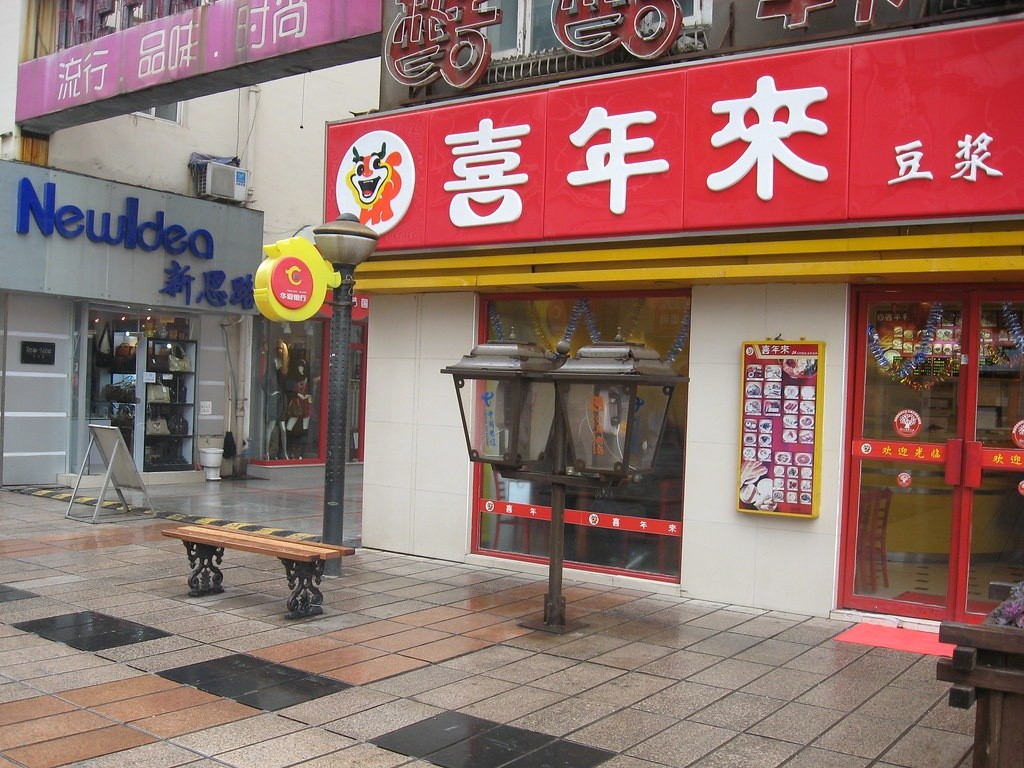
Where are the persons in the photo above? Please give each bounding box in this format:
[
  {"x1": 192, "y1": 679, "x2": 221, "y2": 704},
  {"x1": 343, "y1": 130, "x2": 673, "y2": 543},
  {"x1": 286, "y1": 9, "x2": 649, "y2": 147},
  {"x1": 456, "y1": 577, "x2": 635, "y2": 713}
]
[
  {"x1": 261, "y1": 339, "x2": 290, "y2": 461},
  {"x1": 286, "y1": 357, "x2": 313, "y2": 460}
]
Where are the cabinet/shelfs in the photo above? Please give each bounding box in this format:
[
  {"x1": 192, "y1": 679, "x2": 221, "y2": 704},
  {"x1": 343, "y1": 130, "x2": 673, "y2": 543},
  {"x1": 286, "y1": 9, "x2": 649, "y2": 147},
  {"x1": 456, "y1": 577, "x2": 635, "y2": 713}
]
[
  {"x1": 111, "y1": 331, "x2": 139, "y2": 459},
  {"x1": 143, "y1": 336, "x2": 198, "y2": 472}
]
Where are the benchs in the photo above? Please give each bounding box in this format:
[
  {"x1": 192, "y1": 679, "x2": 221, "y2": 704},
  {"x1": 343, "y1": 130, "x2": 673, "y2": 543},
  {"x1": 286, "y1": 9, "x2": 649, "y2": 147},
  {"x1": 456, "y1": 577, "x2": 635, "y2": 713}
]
[{"x1": 162, "y1": 525, "x2": 355, "y2": 621}]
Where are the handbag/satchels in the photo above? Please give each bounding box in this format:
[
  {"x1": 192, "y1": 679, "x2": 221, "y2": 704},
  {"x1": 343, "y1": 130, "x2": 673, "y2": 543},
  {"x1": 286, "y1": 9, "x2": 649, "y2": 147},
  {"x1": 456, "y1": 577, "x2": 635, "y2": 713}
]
[
  {"x1": 146, "y1": 373, "x2": 187, "y2": 404},
  {"x1": 146, "y1": 404, "x2": 188, "y2": 435},
  {"x1": 111, "y1": 406, "x2": 134, "y2": 429},
  {"x1": 112, "y1": 342, "x2": 133, "y2": 373},
  {"x1": 106, "y1": 376, "x2": 136, "y2": 402},
  {"x1": 148, "y1": 343, "x2": 191, "y2": 372},
  {"x1": 95, "y1": 321, "x2": 114, "y2": 368}
]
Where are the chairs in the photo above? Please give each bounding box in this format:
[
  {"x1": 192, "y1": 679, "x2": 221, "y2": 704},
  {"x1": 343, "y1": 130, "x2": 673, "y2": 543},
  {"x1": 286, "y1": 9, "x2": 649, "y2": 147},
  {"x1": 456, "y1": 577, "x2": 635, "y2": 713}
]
[
  {"x1": 854, "y1": 494, "x2": 868, "y2": 593},
  {"x1": 621, "y1": 479, "x2": 682, "y2": 574},
  {"x1": 867, "y1": 490, "x2": 895, "y2": 593},
  {"x1": 492, "y1": 462, "x2": 542, "y2": 554}
]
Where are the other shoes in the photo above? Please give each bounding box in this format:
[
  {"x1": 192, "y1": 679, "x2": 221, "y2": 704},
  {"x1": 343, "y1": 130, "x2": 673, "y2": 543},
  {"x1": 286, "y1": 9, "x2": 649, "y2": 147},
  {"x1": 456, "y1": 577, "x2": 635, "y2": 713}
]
[{"x1": 151, "y1": 455, "x2": 188, "y2": 465}]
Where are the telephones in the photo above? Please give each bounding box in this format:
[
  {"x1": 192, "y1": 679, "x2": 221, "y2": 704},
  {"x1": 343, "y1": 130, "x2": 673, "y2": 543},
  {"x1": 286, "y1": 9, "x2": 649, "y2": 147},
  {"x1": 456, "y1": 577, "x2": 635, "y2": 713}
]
[
  {"x1": 495, "y1": 378, "x2": 512, "y2": 432},
  {"x1": 592, "y1": 384, "x2": 625, "y2": 438}
]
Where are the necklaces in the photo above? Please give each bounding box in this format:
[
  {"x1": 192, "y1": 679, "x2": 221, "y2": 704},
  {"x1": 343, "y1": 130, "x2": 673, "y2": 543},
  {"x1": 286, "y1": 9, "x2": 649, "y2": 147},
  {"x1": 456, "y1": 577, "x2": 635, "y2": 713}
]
[{"x1": 297, "y1": 377, "x2": 307, "y2": 393}]
